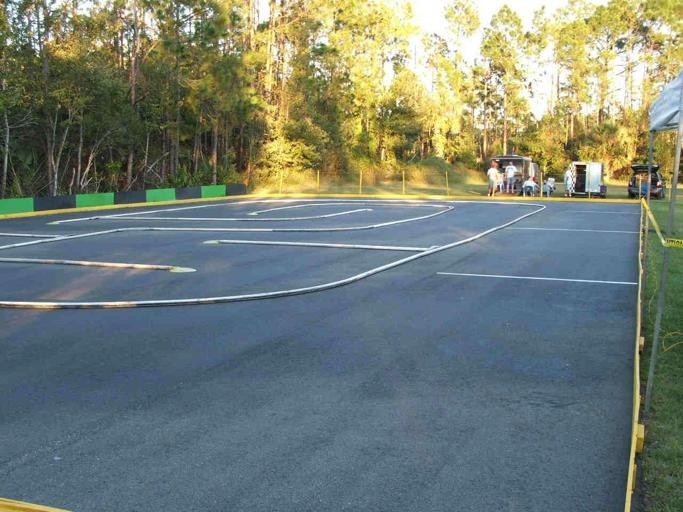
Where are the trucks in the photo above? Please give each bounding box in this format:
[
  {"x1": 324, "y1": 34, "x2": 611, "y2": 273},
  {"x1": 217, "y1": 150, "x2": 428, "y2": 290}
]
[{"x1": 490, "y1": 153, "x2": 540, "y2": 194}]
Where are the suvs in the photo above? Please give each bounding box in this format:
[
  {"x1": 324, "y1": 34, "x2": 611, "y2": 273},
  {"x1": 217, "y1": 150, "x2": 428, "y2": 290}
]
[{"x1": 626, "y1": 162, "x2": 667, "y2": 200}]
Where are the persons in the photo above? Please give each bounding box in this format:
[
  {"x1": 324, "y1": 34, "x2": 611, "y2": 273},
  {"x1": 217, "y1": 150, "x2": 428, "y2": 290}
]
[
  {"x1": 563, "y1": 166, "x2": 575, "y2": 199},
  {"x1": 485, "y1": 162, "x2": 499, "y2": 198},
  {"x1": 504, "y1": 161, "x2": 518, "y2": 194},
  {"x1": 521, "y1": 177, "x2": 539, "y2": 197}
]
